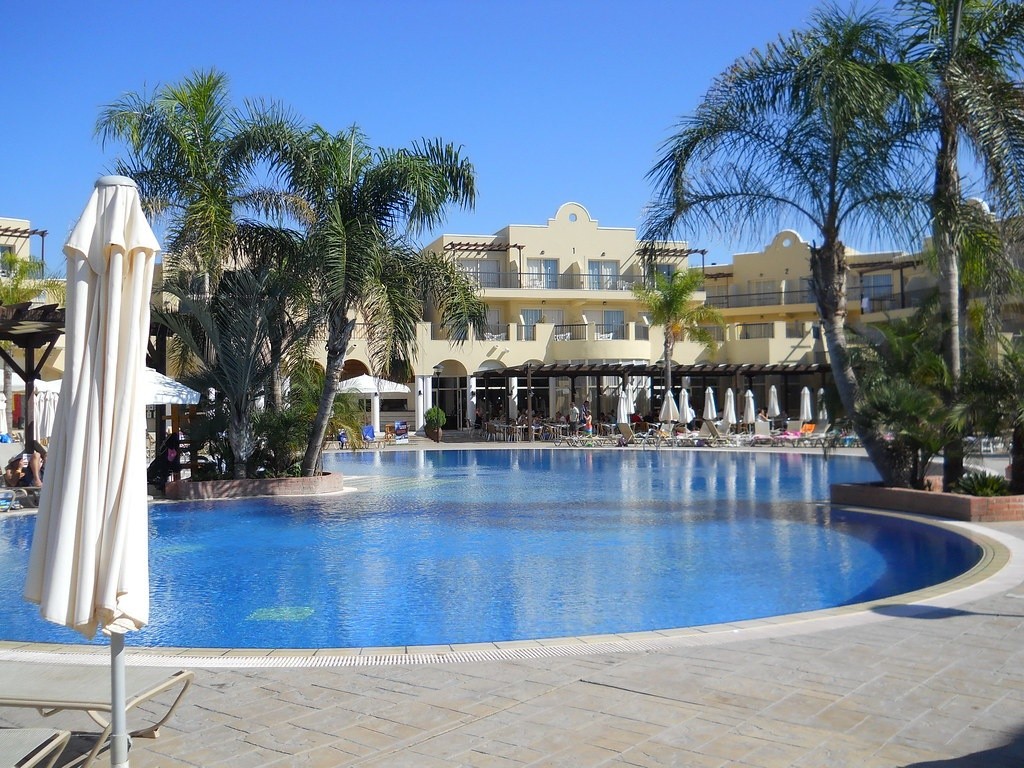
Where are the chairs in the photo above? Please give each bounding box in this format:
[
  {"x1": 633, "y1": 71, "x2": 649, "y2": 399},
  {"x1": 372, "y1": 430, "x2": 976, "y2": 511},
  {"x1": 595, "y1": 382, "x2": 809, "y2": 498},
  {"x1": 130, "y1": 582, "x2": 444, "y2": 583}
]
[{"x1": 466, "y1": 408, "x2": 1012, "y2": 459}]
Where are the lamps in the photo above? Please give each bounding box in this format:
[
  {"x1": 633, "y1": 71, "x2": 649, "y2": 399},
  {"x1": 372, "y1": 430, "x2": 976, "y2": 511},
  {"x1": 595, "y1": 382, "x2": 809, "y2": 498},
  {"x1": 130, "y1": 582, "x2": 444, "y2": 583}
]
[
  {"x1": 419, "y1": 391, "x2": 422, "y2": 395},
  {"x1": 471, "y1": 391, "x2": 475, "y2": 395},
  {"x1": 541, "y1": 250, "x2": 545, "y2": 254},
  {"x1": 601, "y1": 252, "x2": 605, "y2": 256},
  {"x1": 542, "y1": 300, "x2": 546, "y2": 305},
  {"x1": 375, "y1": 392, "x2": 378, "y2": 396},
  {"x1": 603, "y1": 301, "x2": 607, "y2": 305}
]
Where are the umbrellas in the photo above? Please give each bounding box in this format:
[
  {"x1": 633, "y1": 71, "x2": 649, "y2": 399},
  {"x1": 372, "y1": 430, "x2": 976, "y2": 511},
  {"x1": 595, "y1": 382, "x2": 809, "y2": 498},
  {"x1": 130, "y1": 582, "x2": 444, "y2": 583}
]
[
  {"x1": 335, "y1": 373, "x2": 411, "y2": 449},
  {"x1": 0, "y1": 366, "x2": 201, "y2": 406}
]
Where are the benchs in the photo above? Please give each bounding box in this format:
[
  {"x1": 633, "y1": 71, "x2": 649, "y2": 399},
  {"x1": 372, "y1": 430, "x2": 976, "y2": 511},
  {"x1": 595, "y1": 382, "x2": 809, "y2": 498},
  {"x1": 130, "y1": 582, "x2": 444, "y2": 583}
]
[
  {"x1": 361, "y1": 425, "x2": 387, "y2": 449},
  {"x1": 338, "y1": 428, "x2": 350, "y2": 449},
  {"x1": 0, "y1": 670, "x2": 196, "y2": 767},
  {"x1": 0, "y1": 475, "x2": 41, "y2": 513}
]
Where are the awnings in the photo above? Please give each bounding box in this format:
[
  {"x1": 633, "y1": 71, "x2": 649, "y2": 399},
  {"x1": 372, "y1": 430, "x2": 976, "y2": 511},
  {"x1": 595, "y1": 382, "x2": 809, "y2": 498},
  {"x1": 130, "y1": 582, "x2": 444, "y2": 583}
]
[{"x1": 472, "y1": 360, "x2": 864, "y2": 436}]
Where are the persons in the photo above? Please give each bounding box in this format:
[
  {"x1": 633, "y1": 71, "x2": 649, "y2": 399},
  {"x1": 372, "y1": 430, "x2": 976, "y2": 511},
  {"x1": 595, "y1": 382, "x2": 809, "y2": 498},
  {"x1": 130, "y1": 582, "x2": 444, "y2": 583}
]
[
  {"x1": 476, "y1": 400, "x2": 858, "y2": 449},
  {"x1": 4, "y1": 451, "x2": 45, "y2": 489}
]
